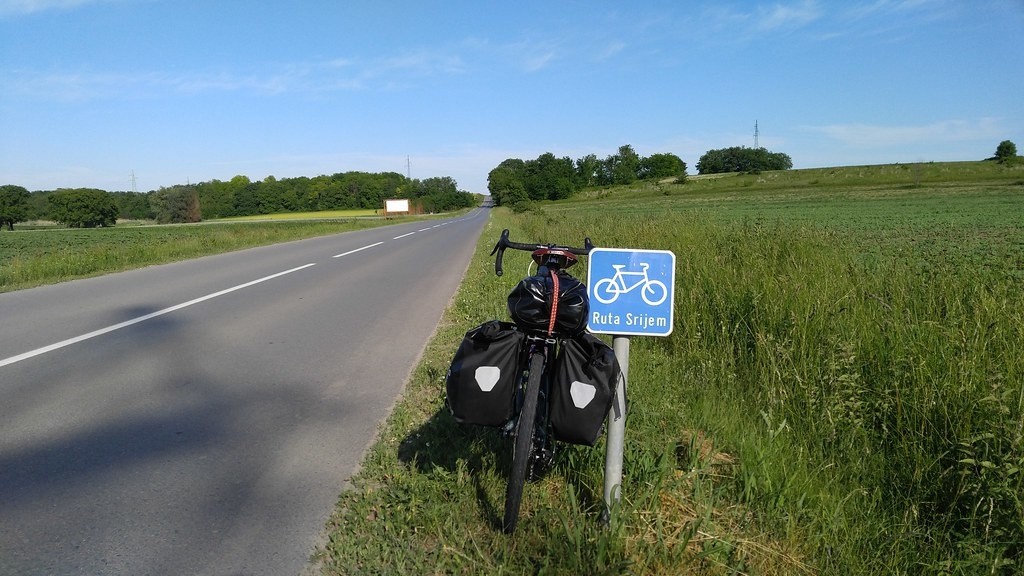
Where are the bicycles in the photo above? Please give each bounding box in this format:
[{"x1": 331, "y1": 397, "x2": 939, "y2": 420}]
[
  {"x1": 484, "y1": 230, "x2": 601, "y2": 535},
  {"x1": 594, "y1": 260, "x2": 668, "y2": 308}
]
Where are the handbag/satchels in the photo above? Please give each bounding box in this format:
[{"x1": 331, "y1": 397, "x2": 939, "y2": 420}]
[
  {"x1": 508, "y1": 272, "x2": 591, "y2": 336},
  {"x1": 444, "y1": 321, "x2": 520, "y2": 427},
  {"x1": 548, "y1": 329, "x2": 623, "y2": 448}
]
